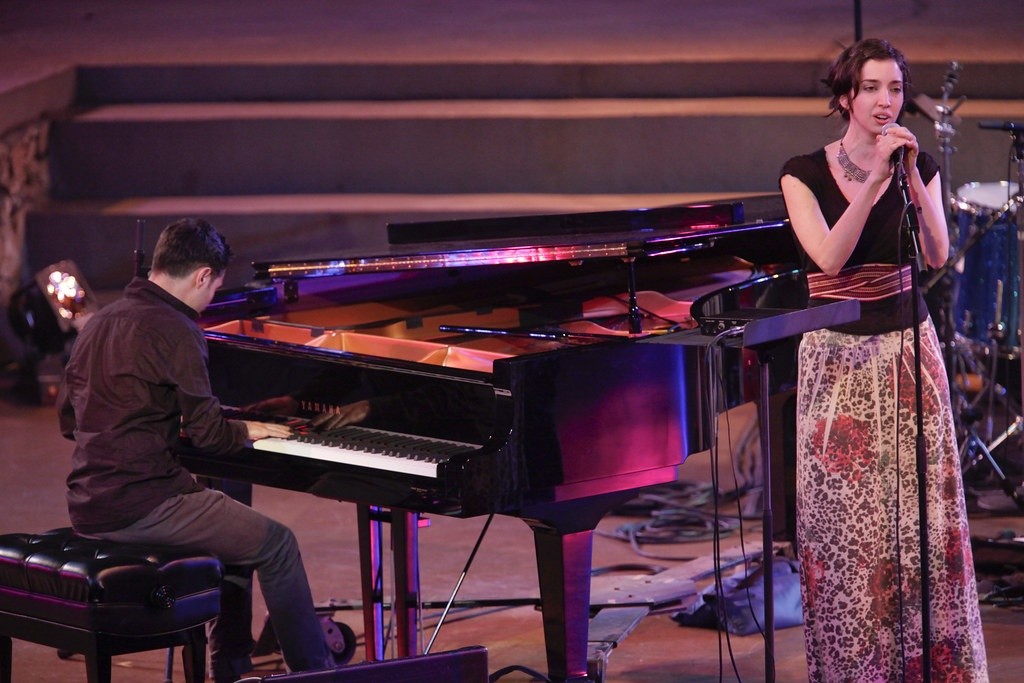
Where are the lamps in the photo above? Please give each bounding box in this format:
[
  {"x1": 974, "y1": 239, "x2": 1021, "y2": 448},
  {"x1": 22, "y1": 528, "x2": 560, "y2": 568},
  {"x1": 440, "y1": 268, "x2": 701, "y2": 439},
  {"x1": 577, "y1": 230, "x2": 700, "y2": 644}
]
[{"x1": 6, "y1": 257, "x2": 103, "y2": 355}]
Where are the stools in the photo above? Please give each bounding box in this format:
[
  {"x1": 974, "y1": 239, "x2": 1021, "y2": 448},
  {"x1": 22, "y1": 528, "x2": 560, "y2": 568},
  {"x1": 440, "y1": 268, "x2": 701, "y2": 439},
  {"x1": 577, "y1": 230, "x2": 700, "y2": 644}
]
[{"x1": 0, "y1": 526, "x2": 227, "y2": 683}]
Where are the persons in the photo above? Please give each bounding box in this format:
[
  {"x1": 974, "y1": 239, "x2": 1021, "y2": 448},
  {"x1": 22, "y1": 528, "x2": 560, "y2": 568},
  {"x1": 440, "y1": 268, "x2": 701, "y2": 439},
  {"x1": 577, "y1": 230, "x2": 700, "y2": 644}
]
[
  {"x1": 55, "y1": 212, "x2": 344, "y2": 671},
  {"x1": 241, "y1": 386, "x2": 379, "y2": 435},
  {"x1": 774, "y1": 40, "x2": 991, "y2": 683}
]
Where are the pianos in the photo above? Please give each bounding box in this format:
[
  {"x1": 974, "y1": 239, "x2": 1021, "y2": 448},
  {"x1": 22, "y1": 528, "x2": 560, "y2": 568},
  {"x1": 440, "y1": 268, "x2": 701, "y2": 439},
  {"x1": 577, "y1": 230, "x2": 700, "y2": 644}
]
[{"x1": 175, "y1": 221, "x2": 800, "y2": 683}]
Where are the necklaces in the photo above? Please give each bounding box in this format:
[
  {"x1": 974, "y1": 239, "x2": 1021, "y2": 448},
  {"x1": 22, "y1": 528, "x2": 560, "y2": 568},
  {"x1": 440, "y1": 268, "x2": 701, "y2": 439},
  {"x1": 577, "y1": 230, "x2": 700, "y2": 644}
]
[{"x1": 837, "y1": 137, "x2": 872, "y2": 183}]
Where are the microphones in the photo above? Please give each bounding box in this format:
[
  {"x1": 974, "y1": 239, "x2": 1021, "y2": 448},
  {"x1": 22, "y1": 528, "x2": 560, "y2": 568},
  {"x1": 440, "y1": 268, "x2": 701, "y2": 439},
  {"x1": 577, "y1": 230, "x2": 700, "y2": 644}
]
[
  {"x1": 978, "y1": 119, "x2": 1024, "y2": 131},
  {"x1": 881, "y1": 122, "x2": 905, "y2": 178}
]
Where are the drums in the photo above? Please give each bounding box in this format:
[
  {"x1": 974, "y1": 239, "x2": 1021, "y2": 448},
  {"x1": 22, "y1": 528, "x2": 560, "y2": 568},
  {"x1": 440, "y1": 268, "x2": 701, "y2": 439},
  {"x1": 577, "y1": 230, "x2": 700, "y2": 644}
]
[{"x1": 953, "y1": 181, "x2": 1023, "y2": 356}]
